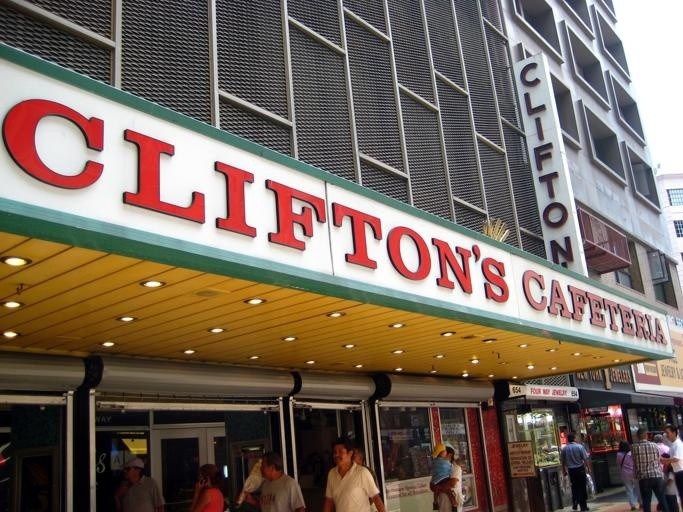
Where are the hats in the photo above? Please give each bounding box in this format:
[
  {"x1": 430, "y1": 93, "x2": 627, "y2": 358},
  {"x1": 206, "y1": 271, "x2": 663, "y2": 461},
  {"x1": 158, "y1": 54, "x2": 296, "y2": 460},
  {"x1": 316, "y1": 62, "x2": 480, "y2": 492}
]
[{"x1": 124, "y1": 458, "x2": 144, "y2": 468}]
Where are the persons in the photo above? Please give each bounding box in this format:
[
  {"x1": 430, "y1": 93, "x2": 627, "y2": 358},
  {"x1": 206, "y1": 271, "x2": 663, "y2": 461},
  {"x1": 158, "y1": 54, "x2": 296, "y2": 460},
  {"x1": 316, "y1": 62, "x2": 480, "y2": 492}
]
[
  {"x1": 322, "y1": 437, "x2": 386, "y2": 512},
  {"x1": 114, "y1": 458, "x2": 166, "y2": 511},
  {"x1": 352, "y1": 446, "x2": 379, "y2": 511},
  {"x1": 560, "y1": 432, "x2": 591, "y2": 511},
  {"x1": 430, "y1": 445, "x2": 462, "y2": 512},
  {"x1": 617, "y1": 424, "x2": 683, "y2": 512},
  {"x1": 431, "y1": 444, "x2": 459, "y2": 512},
  {"x1": 190, "y1": 464, "x2": 225, "y2": 512},
  {"x1": 242, "y1": 452, "x2": 306, "y2": 512}
]
